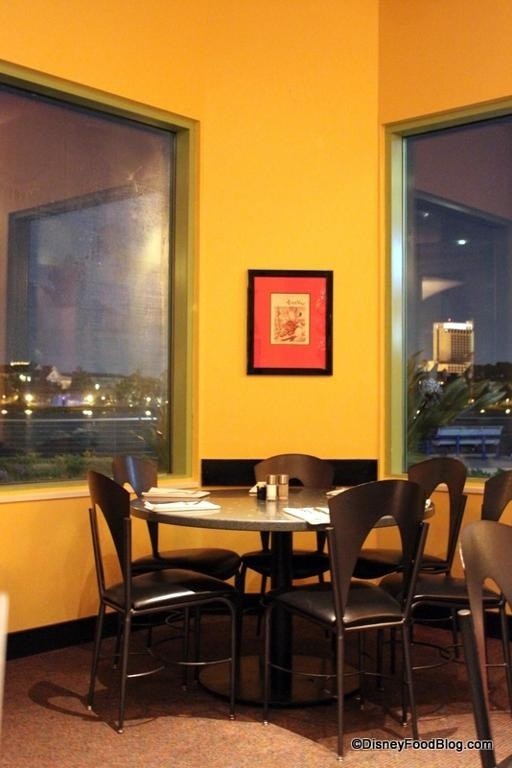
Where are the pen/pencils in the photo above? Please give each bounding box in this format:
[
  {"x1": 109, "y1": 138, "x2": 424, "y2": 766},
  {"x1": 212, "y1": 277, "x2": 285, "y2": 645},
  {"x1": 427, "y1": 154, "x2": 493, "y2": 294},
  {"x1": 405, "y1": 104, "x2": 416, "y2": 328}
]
[
  {"x1": 194, "y1": 499, "x2": 203, "y2": 505},
  {"x1": 314, "y1": 508, "x2": 329, "y2": 515}
]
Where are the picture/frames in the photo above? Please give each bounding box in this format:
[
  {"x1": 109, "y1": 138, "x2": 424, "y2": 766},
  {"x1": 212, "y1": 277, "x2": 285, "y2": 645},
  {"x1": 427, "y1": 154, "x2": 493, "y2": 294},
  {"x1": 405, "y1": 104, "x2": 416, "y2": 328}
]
[{"x1": 244, "y1": 265, "x2": 334, "y2": 381}]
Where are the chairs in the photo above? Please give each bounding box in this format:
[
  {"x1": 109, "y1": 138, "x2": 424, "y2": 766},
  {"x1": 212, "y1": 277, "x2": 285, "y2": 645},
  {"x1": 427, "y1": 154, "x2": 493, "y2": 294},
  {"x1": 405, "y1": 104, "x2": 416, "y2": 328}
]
[{"x1": 73, "y1": 452, "x2": 512, "y2": 768}]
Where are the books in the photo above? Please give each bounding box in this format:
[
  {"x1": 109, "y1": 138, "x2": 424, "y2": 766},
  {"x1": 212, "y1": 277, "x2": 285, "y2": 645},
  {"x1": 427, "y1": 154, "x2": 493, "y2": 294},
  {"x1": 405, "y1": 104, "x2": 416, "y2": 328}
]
[
  {"x1": 282, "y1": 506, "x2": 331, "y2": 525},
  {"x1": 139, "y1": 485, "x2": 213, "y2": 499},
  {"x1": 142, "y1": 499, "x2": 221, "y2": 513}
]
